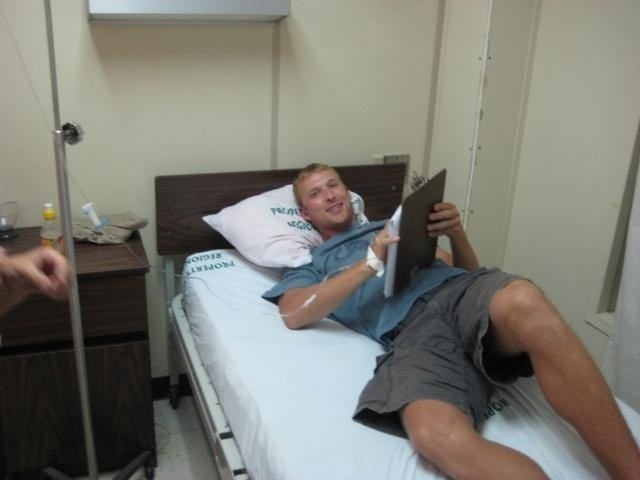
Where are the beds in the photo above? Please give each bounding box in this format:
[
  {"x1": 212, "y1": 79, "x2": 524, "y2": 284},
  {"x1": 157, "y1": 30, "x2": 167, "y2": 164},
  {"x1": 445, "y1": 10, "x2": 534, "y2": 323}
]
[{"x1": 153, "y1": 163, "x2": 640, "y2": 480}]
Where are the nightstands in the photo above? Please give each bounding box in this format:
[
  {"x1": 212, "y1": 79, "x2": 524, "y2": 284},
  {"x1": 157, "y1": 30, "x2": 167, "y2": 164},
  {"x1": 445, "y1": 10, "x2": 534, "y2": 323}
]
[{"x1": 0, "y1": 225, "x2": 157, "y2": 473}]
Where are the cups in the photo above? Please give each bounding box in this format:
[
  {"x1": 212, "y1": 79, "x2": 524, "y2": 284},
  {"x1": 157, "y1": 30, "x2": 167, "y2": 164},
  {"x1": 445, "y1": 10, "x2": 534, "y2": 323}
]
[{"x1": 0, "y1": 202, "x2": 16, "y2": 239}]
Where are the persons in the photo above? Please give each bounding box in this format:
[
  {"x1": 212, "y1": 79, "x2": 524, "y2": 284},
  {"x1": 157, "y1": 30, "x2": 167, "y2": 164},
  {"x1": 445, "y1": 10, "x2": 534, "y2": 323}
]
[
  {"x1": 262, "y1": 164, "x2": 640, "y2": 480},
  {"x1": 0, "y1": 245, "x2": 73, "y2": 319}
]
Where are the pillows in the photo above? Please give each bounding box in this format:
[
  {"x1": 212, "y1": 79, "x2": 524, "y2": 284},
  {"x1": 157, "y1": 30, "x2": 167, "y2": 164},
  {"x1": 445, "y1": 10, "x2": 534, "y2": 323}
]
[{"x1": 199, "y1": 183, "x2": 370, "y2": 269}]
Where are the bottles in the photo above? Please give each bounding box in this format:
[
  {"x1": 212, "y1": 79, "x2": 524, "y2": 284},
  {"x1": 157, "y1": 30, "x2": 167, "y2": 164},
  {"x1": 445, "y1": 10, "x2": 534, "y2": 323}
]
[{"x1": 40, "y1": 202, "x2": 63, "y2": 254}]
